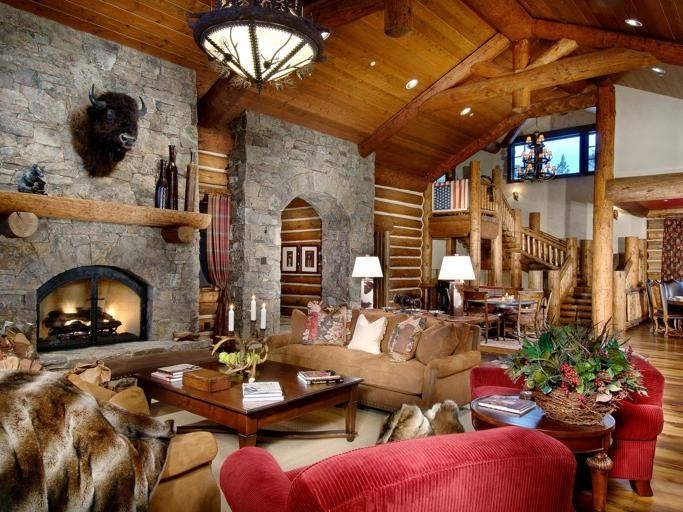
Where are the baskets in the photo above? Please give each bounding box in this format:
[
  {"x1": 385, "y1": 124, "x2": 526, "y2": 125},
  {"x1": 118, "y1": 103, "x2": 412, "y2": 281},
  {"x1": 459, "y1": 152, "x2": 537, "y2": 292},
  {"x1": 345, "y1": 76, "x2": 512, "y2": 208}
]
[{"x1": 534, "y1": 387, "x2": 618, "y2": 426}]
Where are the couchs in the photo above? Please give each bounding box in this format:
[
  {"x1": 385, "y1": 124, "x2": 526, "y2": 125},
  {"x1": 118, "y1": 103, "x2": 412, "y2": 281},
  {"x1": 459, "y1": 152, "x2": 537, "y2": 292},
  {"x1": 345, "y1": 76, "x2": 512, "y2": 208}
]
[{"x1": 267, "y1": 309, "x2": 481, "y2": 414}]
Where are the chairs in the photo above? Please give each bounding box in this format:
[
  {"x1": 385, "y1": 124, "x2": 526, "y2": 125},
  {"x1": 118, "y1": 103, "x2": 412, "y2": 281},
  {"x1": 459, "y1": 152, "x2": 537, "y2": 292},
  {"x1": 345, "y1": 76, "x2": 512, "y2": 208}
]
[
  {"x1": 0, "y1": 373, "x2": 221, "y2": 512},
  {"x1": 464, "y1": 288, "x2": 553, "y2": 345},
  {"x1": 646, "y1": 279, "x2": 683, "y2": 338},
  {"x1": 219, "y1": 426, "x2": 578, "y2": 512},
  {"x1": 470, "y1": 352, "x2": 665, "y2": 497}
]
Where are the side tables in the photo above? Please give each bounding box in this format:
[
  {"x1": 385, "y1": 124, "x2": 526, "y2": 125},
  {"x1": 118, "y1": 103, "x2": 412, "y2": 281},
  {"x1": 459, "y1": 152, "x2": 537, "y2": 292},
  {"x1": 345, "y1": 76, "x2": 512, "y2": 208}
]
[{"x1": 470, "y1": 393, "x2": 617, "y2": 512}]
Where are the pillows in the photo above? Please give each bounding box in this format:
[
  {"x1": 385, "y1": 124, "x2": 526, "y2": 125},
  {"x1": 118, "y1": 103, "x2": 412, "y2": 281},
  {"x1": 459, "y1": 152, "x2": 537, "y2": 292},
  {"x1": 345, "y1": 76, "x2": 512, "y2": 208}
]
[
  {"x1": 288, "y1": 300, "x2": 459, "y2": 366},
  {"x1": 65, "y1": 374, "x2": 151, "y2": 418},
  {"x1": 376, "y1": 400, "x2": 465, "y2": 444}
]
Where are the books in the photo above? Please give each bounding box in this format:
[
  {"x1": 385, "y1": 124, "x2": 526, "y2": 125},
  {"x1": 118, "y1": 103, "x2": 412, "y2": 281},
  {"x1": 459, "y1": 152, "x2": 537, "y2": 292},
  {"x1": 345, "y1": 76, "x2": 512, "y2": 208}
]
[
  {"x1": 152, "y1": 362, "x2": 201, "y2": 382},
  {"x1": 478, "y1": 393, "x2": 536, "y2": 416},
  {"x1": 240, "y1": 368, "x2": 344, "y2": 404}
]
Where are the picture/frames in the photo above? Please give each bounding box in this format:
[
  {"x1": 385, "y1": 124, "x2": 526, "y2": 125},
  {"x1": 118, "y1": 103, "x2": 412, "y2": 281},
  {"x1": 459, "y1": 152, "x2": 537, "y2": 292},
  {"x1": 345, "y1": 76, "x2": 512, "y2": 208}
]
[
  {"x1": 300, "y1": 245, "x2": 319, "y2": 274},
  {"x1": 281, "y1": 245, "x2": 298, "y2": 273}
]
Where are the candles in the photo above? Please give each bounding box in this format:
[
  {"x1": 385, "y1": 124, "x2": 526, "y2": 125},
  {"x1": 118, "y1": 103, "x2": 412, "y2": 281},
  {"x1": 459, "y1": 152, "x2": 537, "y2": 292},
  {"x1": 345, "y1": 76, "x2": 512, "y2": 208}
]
[
  {"x1": 228, "y1": 303, "x2": 235, "y2": 331},
  {"x1": 260, "y1": 301, "x2": 267, "y2": 329},
  {"x1": 250, "y1": 294, "x2": 257, "y2": 321}
]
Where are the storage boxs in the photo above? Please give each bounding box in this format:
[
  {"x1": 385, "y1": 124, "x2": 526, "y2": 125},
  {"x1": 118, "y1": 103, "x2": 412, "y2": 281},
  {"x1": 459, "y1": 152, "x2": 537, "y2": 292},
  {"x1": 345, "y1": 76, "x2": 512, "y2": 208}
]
[{"x1": 183, "y1": 368, "x2": 231, "y2": 393}]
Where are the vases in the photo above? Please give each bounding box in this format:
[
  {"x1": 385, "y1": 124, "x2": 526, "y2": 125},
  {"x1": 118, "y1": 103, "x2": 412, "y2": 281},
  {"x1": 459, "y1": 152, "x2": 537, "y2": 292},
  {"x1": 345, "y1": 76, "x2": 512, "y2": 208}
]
[
  {"x1": 155, "y1": 159, "x2": 169, "y2": 209},
  {"x1": 184, "y1": 152, "x2": 198, "y2": 211},
  {"x1": 166, "y1": 145, "x2": 178, "y2": 210}
]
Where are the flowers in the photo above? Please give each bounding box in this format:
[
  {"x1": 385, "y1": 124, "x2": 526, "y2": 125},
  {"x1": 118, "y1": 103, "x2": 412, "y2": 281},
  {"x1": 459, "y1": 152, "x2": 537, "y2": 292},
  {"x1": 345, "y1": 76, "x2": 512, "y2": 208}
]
[{"x1": 502, "y1": 316, "x2": 649, "y2": 407}]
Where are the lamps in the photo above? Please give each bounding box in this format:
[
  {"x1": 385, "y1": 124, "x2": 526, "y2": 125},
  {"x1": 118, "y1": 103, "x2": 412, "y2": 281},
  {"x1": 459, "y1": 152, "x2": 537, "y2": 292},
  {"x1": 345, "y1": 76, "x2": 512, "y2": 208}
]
[
  {"x1": 194, "y1": 0, "x2": 331, "y2": 92},
  {"x1": 438, "y1": 255, "x2": 476, "y2": 316},
  {"x1": 517, "y1": 117, "x2": 556, "y2": 183},
  {"x1": 352, "y1": 254, "x2": 384, "y2": 310}
]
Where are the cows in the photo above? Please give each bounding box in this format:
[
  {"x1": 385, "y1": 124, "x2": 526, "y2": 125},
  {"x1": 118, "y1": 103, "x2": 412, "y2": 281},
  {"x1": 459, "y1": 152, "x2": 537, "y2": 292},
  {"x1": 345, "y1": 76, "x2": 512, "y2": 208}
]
[{"x1": 68, "y1": 81, "x2": 149, "y2": 179}]
